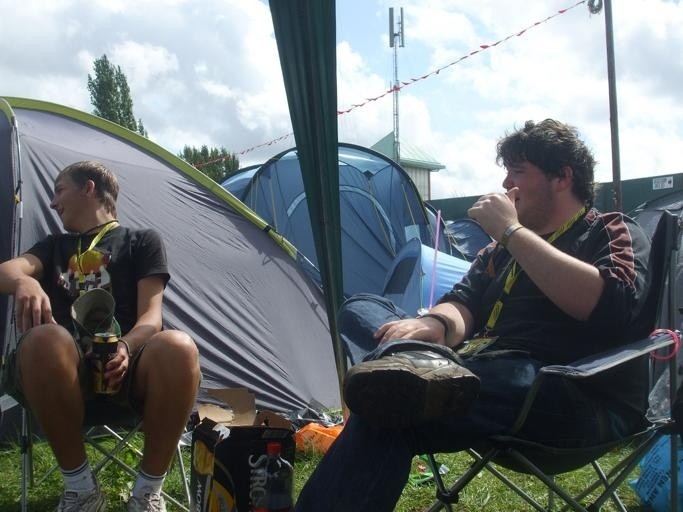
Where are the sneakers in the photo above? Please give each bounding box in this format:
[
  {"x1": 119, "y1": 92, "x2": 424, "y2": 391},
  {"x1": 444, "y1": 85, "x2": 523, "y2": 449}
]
[
  {"x1": 340, "y1": 346, "x2": 485, "y2": 423},
  {"x1": 127, "y1": 488, "x2": 169, "y2": 512},
  {"x1": 57, "y1": 472, "x2": 108, "y2": 511}
]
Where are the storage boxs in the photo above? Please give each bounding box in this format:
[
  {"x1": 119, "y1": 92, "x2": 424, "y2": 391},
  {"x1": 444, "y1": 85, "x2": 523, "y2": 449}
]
[{"x1": 191, "y1": 384, "x2": 296, "y2": 510}]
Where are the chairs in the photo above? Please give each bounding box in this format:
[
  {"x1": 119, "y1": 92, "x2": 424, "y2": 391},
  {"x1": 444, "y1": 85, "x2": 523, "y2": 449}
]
[
  {"x1": 409, "y1": 209, "x2": 683, "y2": 512},
  {"x1": 2, "y1": 284, "x2": 197, "y2": 512}
]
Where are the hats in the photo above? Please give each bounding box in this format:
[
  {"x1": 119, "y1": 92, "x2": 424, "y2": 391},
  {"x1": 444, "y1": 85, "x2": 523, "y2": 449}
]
[{"x1": 68, "y1": 287, "x2": 123, "y2": 355}]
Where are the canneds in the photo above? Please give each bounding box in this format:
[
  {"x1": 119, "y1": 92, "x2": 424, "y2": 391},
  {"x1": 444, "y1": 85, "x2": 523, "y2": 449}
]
[{"x1": 92, "y1": 333, "x2": 121, "y2": 394}]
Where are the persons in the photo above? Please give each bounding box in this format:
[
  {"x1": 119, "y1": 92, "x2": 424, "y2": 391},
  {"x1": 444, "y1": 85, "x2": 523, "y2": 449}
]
[
  {"x1": 292, "y1": 120, "x2": 654, "y2": 512},
  {"x1": 0, "y1": 161, "x2": 202, "y2": 512}
]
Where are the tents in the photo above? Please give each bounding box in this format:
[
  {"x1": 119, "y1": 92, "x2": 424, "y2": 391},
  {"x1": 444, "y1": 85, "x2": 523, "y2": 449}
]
[{"x1": 0, "y1": 95, "x2": 349, "y2": 434}]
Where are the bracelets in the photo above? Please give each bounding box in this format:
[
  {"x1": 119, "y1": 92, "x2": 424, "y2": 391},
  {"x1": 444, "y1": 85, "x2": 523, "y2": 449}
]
[
  {"x1": 415, "y1": 314, "x2": 449, "y2": 338},
  {"x1": 502, "y1": 223, "x2": 523, "y2": 250},
  {"x1": 117, "y1": 337, "x2": 131, "y2": 357}
]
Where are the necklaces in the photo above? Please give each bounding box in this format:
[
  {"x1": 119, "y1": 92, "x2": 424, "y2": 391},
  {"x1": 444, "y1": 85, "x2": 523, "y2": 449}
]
[{"x1": 78, "y1": 219, "x2": 119, "y2": 236}]
